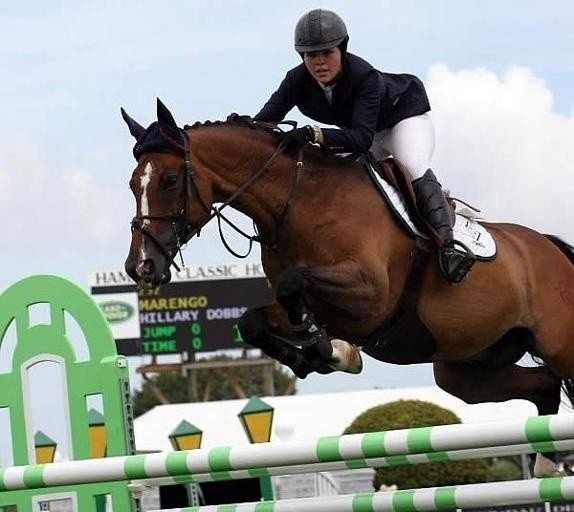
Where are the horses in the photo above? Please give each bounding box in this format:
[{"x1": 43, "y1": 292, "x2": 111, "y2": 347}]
[{"x1": 119, "y1": 95, "x2": 574, "y2": 480}]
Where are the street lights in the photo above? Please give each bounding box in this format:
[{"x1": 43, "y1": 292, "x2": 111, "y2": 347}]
[
  {"x1": 34, "y1": 430, "x2": 58, "y2": 465},
  {"x1": 236, "y1": 394, "x2": 275, "y2": 501},
  {"x1": 87, "y1": 407, "x2": 107, "y2": 512},
  {"x1": 169, "y1": 419, "x2": 203, "y2": 451}
]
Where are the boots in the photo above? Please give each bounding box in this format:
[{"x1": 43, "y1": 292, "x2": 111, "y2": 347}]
[{"x1": 410, "y1": 167, "x2": 465, "y2": 275}]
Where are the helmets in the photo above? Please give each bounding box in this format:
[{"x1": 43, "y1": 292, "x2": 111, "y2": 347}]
[{"x1": 293, "y1": 8, "x2": 348, "y2": 54}]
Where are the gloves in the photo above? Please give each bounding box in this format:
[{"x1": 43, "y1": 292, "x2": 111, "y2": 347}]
[{"x1": 288, "y1": 126, "x2": 315, "y2": 143}]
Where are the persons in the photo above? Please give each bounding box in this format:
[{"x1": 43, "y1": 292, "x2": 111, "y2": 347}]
[{"x1": 252, "y1": 9, "x2": 473, "y2": 289}]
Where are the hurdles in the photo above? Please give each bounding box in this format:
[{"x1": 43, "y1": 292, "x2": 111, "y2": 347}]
[{"x1": 0, "y1": 275, "x2": 574, "y2": 512}]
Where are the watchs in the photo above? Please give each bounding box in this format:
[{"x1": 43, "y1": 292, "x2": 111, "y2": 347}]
[{"x1": 311, "y1": 123, "x2": 322, "y2": 144}]
